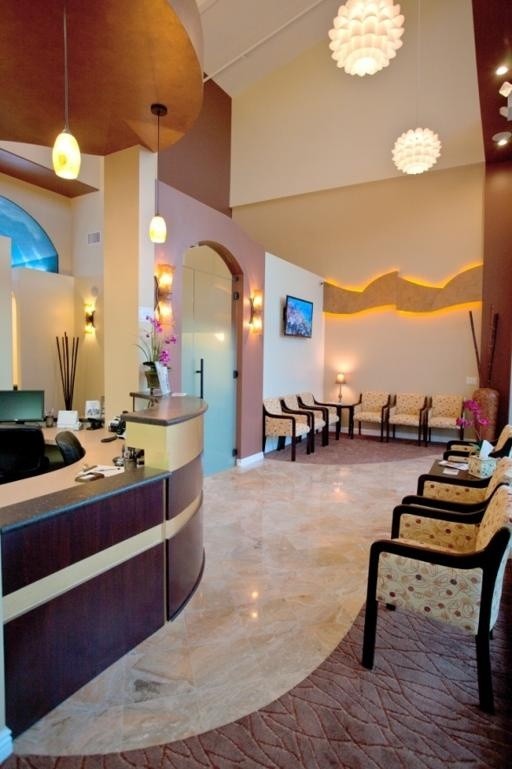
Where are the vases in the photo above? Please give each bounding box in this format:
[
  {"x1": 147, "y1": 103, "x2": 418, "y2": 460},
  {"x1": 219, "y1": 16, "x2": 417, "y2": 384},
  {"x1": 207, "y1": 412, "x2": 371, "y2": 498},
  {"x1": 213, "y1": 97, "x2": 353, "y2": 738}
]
[
  {"x1": 142, "y1": 362, "x2": 161, "y2": 389},
  {"x1": 472, "y1": 386, "x2": 501, "y2": 443}
]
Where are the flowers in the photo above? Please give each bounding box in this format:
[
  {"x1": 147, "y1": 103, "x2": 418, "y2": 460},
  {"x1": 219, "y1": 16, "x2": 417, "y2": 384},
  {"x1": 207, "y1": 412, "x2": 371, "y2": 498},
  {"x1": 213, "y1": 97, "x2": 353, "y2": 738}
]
[
  {"x1": 135, "y1": 315, "x2": 176, "y2": 367},
  {"x1": 455, "y1": 399, "x2": 490, "y2": 443}
]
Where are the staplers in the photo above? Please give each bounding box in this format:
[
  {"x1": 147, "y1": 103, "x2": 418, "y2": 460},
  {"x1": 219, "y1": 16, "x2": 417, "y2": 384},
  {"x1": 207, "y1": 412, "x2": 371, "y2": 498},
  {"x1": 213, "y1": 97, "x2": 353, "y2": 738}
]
[{"x1": 86, "y1": 418, "x2": 103, "y2": 429}]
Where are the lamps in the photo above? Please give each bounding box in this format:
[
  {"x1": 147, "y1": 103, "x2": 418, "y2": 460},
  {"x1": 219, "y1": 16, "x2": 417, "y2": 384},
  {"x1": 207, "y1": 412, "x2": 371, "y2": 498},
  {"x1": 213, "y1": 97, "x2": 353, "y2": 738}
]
[
  {"x1": 248, "y1": 288, "x2": 264, "y2": 333},
  {"x1": 390, "y1": 0, "x2": 442, "y2": 176},
  {"x1": 334, "y1": 372, "x2": 347, "y2": 404},
  {"x1": 51, "y1": 0, "x2": 81, "y2": 180},
  {"x1": 149, "y1": 103, "x2": 168, "y2": 244},
  {"x1": 328, "y1": 0, "x2": 405, "y2": 79},
  {"x1": 83, "y1": 295, "x2": 98, "y2": 340},
  {"x1": 153, "y1": 263, "x2": 176, "y2": 332}
]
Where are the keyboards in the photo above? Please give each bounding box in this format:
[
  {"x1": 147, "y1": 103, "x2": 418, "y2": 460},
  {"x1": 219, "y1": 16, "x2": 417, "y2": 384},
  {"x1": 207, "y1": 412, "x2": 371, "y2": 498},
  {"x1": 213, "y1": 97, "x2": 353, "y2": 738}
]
[{"x1": 0, "y1": 424, "x2": 41, "y2": 431}]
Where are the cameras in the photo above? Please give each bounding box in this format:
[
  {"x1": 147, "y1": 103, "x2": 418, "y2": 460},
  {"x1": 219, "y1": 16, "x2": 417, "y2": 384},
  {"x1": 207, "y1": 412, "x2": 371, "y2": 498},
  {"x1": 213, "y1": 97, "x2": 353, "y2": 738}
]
[{"x1": 108, "y1": 410, "x2": 128, "y2": 435}]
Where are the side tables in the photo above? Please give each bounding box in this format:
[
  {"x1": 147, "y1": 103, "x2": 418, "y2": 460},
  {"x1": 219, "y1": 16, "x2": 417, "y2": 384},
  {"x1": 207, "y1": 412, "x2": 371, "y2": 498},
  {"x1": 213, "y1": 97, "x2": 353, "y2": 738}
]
[
  {"x1": 427, "y1": 458, "x2": 479, "y2": 482},
  {"x1": 320, "y1": 402, "x2": 358, "y2": 437}
]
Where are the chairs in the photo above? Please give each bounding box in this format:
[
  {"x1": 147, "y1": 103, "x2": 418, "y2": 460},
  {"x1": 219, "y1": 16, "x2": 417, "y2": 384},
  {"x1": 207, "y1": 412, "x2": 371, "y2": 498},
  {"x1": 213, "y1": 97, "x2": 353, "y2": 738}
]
[
  {"x1": 401, "y1": 456, "x2": 512, "y2": 515},
  {"x1": 54, "y1": 430, "x2": 84, "y2": 465},
  {"x1": 423, "y1": 394, "x2": 466, "y2": 448},
  {"x1": 0, "y1": 423, "x2": 53, "y2": 485},
  {"x1": 385, "y1": 392, "x2": 429, "y2": 446},
  {"x1": 264, "y1": 391, "x2": 341, "y2": 462},
  {"x1": 444, "y1": 422, "x2": 512, "y2": 461},
  {"x1": 349, "y1": 391, "x2": 391, "y2": 442},
  {"x1": 361, "y1": 483, "x2": 512, "y2": 715}
]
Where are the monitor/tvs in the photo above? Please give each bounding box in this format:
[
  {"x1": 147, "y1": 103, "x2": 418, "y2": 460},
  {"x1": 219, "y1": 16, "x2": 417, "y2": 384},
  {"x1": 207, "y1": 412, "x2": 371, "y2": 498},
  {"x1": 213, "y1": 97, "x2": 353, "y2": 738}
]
[
  {"x1": 284, "y1": 295, "x2": 313, "y2": 337},
  {"x1": 0, "y1": 390, "x2": 44, "y2": 424}
]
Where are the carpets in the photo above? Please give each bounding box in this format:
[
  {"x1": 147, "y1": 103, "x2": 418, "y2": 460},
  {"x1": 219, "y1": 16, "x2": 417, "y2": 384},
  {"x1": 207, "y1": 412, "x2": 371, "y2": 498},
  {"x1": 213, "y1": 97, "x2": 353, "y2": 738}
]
[
  {"x1": 264, "y1": 431, "x2": 448, "y2": 465},
  {"x1": 0, "y1": 556, "x2": 512, "y2": 769}
]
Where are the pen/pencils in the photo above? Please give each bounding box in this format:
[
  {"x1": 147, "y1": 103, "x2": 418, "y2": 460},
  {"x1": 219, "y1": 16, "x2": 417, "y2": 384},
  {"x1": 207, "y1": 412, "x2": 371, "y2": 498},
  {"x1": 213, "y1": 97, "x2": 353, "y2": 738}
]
[
  {"x1": 98, "y1": 468, "x2": 120, "y2": 471},
  {"x1": 45, "y1": 408, "x2": 54, "y2": 415}
]
